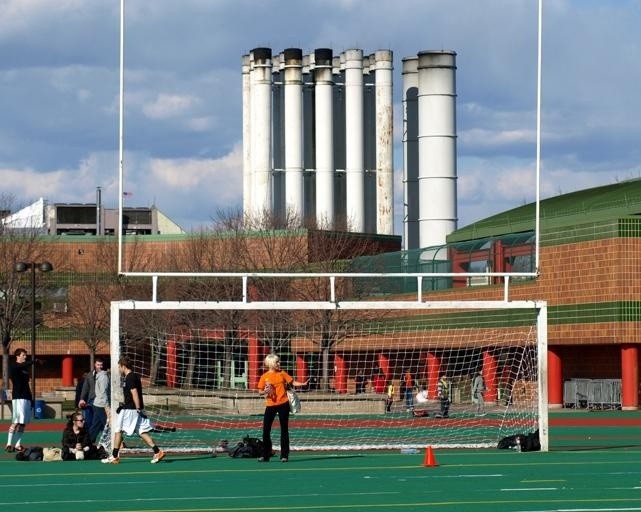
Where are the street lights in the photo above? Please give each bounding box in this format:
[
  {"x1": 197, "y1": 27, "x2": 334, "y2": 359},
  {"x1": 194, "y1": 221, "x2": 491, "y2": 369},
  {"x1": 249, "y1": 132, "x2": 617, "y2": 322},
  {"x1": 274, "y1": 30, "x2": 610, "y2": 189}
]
[{"x1": 14, "y1": 261, "x2": 55, "y2": 420}]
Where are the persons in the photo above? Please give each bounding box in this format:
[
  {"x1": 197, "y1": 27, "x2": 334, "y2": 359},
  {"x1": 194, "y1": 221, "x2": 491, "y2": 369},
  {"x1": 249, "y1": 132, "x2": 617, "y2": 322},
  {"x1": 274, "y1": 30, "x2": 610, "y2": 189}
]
[
  {"x1": 61, "y1": 358, "x2": 127, "y2": 461},
  {"x1": 355, "y1": 365, "x2": 393, "y2": 413},
  {"x1": 5, "y1": 348, "x2": 43, "y2": 452},
  {"x1": 100, "y1": 358, "x2": 166, "y2": 465},
  {"x1": 257, "y1": 354, "x2": 311, "y2": 462},
  {"x1": 437, "y1": 372, "x2": 451, "y2": 418},
  {"x1": 400, "y1": 368, "x2": 415, "y2": 412},
  {"x1": 473, "y1": 368, "x2": 488, "y2": 417}
]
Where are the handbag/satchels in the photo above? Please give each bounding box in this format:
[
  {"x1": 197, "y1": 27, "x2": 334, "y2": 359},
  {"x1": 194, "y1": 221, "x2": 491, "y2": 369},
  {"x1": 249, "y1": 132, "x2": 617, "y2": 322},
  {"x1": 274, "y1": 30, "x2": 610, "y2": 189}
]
[
  {"x1": 228, "y1": 436, "x2": 276, "y2": 458},
  {"x1": 498, "y1": 430, "x2": 541, "y2": 452},
  {"x1": 285, "y1": 389, "x2": 302, "y2": 414}
]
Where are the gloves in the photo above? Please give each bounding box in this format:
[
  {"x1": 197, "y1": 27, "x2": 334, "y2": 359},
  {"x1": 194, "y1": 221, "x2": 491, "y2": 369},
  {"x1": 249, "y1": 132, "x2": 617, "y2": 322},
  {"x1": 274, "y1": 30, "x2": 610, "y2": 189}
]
[
  {"x1": 116, "y1": 406, "x2": 122, "y2": 414},
  {"x1": 137, "y1": 408, "x2": 147, "y2": 419}
]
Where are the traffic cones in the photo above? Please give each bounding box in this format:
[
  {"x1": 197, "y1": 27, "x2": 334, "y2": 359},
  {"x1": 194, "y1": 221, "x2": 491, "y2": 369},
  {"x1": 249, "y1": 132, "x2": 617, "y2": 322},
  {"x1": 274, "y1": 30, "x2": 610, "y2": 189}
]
[{"x1": 420, "y1": 445, "x2": 440, "y2": 466}]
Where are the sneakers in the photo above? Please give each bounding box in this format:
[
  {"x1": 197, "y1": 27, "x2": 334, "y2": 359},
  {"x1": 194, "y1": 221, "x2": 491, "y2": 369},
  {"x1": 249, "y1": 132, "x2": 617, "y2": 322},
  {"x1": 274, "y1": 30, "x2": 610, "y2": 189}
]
[
  {"x1": 258, "y1": 457, "x2": 269, "y2": 462},
  {"x1": 5, "y1": 446, "x2": 26, "y2": 452},
  {"x1": 100, "y1": 454, "x2": 120, "y2": 464},
  {"x1": 281, "y1": 458, "x2": 288, "y2": 462},
  {"x1": 150, "y1": 449, "x2": 166, "y2": 464}
]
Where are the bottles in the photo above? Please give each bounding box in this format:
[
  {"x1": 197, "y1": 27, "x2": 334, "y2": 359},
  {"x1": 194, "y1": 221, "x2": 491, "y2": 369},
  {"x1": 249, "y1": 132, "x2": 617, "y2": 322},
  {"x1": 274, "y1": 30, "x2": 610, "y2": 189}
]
[{"x1": 265, "y1": 378, "x2": 272, "y2": 393}]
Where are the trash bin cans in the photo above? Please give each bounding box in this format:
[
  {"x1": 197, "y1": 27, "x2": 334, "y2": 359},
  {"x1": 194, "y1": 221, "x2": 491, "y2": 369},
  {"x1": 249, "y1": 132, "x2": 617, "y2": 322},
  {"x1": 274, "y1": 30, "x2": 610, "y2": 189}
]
[{"x1": 34, "y1": 400, "x2": 44, "y2": 419}]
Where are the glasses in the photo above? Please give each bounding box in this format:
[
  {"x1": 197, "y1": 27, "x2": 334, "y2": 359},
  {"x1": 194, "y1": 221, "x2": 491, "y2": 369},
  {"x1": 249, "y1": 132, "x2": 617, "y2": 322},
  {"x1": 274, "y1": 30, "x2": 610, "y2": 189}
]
[{"x1": 75, "y1": 418, "x2": 84, "y2": 422}]
